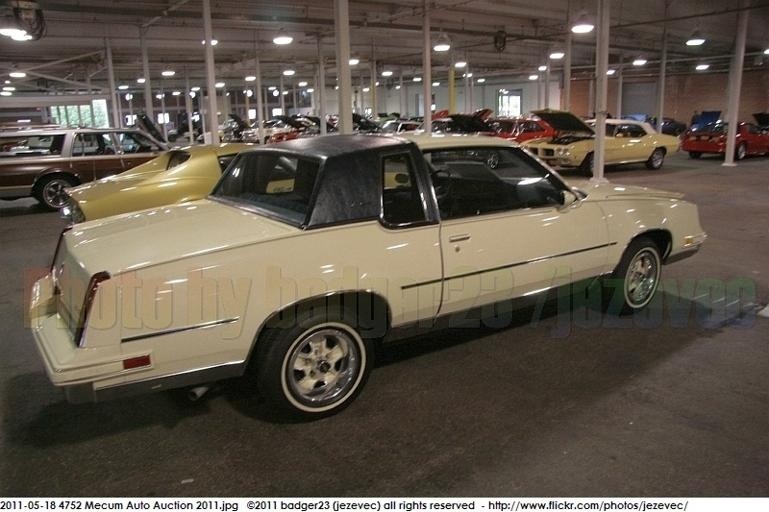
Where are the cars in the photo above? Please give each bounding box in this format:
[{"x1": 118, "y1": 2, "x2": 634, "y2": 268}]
[
  {"x1": 683, "y1": 121, "x2": 769, "y2": 160},
  {"x1": 59, "y1": 143, "x2": 256, "y2": 223},
  {"x1": 28, "y1": 133, "x2": 707, "y2": 421},
  {"x1": 0, "y1": 112, "x2": 170, "y2": 211}
]
